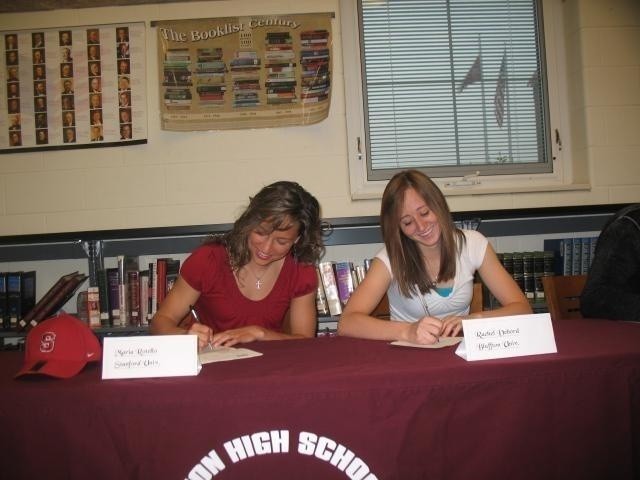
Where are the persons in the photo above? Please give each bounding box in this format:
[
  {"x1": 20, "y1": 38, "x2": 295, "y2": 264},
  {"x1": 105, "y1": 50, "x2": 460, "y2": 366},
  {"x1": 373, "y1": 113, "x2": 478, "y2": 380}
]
[
  {"x1": 3, "y1": 25, "x2": 134, "y2": 147},
  {"x1": 335, "y1": 167, "x2": 536, "y2": 347},
  {"x1": 144, "y1": 180, "x2": 333, "y2": 353},
  {"x1": 580, "y1": 202, "x2": 640, "y2": 324}
]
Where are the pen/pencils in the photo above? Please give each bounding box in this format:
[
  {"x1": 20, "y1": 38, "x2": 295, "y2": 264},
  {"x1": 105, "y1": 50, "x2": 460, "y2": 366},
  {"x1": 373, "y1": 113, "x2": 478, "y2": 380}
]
[
  {"x1": 190, "y1": 305, "x2": 213, "y2": 351},
  {"x1": 414, "y1": 283, "x2": 440, "y2": 342}
]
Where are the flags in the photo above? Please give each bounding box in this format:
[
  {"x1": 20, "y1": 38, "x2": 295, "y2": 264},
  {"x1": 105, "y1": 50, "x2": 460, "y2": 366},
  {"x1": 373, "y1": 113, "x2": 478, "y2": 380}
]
[
  {"x1": 494, "y1": 52, "x2": 507, "y2": 128},
  {"x1": 460, "y1": 54, "x2": 484, "y2": 94}
]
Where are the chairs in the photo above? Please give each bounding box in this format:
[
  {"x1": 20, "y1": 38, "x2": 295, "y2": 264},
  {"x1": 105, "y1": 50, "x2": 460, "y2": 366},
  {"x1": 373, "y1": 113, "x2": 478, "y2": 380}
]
[
  {"x1": 178, "y1": 302, "x2": 291, "y2": 335},
  {"x1": 370, "y1": 283, "x2": 484, "y2": 319},
  {"x1": 542, "y1": 275, "x2": 589, "y2": 319}
]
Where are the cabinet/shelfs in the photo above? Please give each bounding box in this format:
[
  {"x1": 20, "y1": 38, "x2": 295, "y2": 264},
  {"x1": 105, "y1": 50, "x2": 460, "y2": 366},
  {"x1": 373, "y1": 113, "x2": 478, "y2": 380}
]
[{"x1": 0, "y1": 206, "x2": 640, "y2": 350}]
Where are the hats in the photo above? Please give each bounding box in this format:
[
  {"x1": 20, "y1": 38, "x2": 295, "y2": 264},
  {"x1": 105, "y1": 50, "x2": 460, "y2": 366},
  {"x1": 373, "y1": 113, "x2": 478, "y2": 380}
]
[{"x1": 14, "y1": 313, "x2": 101, "y2": 379}]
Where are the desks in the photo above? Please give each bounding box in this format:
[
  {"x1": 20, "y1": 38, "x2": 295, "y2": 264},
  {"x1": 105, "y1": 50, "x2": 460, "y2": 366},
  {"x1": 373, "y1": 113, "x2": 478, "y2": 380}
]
[{"x1": 1, "y1": 316, "x2": 640, "y2": 480}]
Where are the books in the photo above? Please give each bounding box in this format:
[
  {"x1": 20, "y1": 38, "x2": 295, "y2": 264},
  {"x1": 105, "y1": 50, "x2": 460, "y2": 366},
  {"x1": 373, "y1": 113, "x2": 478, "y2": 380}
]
[
  {"x1": 484, "y1": 238, "x2": 600, "y2": 306},
  {"x1": 315, "y1": 258, "x2": 373, "y2": 316},
  {"x1": 1, "y1": 257, "x2": 180, "y2": 335}
]
